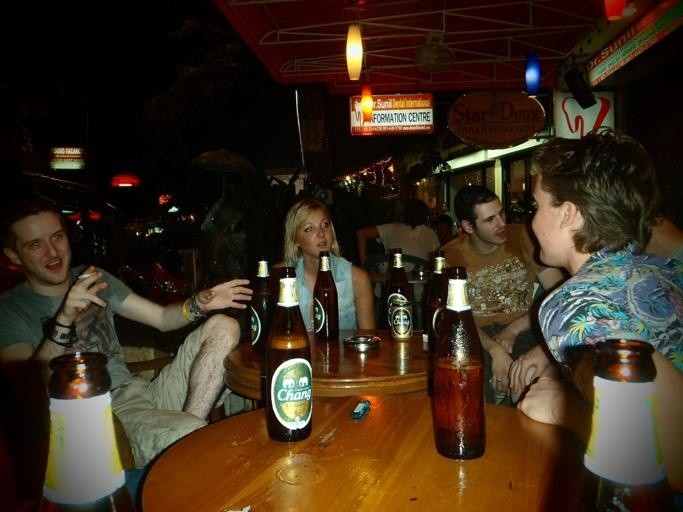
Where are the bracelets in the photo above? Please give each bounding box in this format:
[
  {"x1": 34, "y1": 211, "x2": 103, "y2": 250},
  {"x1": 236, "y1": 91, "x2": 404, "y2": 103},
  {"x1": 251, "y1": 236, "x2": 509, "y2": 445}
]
[
  {"x1": 183, "y1": 294, "x2": 207, "y2": 322},
  {"x1": 48, "y1": 321, "x2": 78, "y2": 347}
]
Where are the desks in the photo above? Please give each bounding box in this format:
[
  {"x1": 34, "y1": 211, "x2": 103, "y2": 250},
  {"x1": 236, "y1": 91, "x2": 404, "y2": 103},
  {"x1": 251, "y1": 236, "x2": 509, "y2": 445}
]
[
  {"x1": 225, "y1": 327, "x2": 486, "y2": 401},
  {"x1": 138, "y1": 394, "x2": 583, "y2": 512}
]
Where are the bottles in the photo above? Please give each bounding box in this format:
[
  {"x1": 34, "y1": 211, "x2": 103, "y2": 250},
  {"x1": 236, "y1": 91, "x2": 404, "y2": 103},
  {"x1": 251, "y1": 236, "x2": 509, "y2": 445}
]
[
  {"x1": 562, "y1": 339, "x2": 676, "y2": 512},
  {"x1": 386, "y1": 249, "x2": 414, "y2": 340},
  {"x1": 312, "y1": 251, "x2": 341, "y2": 344},
  {"x1": 35, "y1": 351, "x2": 134, "y2": 512},
  {"x1": 250, "y1": 255, "x2": 273, "y2": 362},
  {"x1": 265, "y1": 267, "x2": 312, "y2": 441},
  {"x1": 422, "y1": 252, "x2": 449, "y2": 355},
  {"x1": 429, "y1": 268, "x2": 484, "y2": 459}
]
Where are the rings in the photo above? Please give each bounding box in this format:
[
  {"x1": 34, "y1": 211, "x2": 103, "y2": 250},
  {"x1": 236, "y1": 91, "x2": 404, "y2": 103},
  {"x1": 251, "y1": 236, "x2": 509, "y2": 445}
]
[{"x1": 497, "y1": 379, "x2": 503, "y2": 384}]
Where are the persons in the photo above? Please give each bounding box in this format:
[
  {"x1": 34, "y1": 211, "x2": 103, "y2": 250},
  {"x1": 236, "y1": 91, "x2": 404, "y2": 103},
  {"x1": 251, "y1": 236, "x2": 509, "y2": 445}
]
[
  {"x1": 641, "y1": 207, "x2": 683, "y2": 262},
  {"x1": 1, "y1": 197, "x2": 255, "y2": 470},
  {"x1": 431, "y1": 215, "x2": 453, "y2": 247},
  {"x1": 355, "y1": 198, "x2": 440, "y2": 302},
  {"x1": 265, "y1": 194, "x2": 377, "y2": 331},
  {"x1": 433, "y1": 185, "x2": 568, "y2": 408},
  {"x1": 532, "y1": 134, "x2": 682, "y2": 497}
]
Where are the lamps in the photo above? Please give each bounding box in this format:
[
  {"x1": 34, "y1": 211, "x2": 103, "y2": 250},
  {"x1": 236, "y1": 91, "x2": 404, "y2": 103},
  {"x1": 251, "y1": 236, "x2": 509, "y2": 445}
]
[
  {"x1": 603, "y1": 0, "x2": 647, "y2": 21},
  {"x1": 524, "y1": 50, "x2": 541, "y2": 94},
  {"x1": 344, "y1": 12, "x2": 364, "y2": 81}
]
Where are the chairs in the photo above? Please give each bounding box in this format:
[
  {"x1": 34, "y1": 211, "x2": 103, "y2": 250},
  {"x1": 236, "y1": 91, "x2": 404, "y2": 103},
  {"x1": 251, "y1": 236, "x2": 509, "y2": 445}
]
[
  {"x1": 363, "y1": 254, "x2": 435, "y2": 330},
  {"x1": 107, "y1": 357, "x2": 223, "y2": 512}
]
